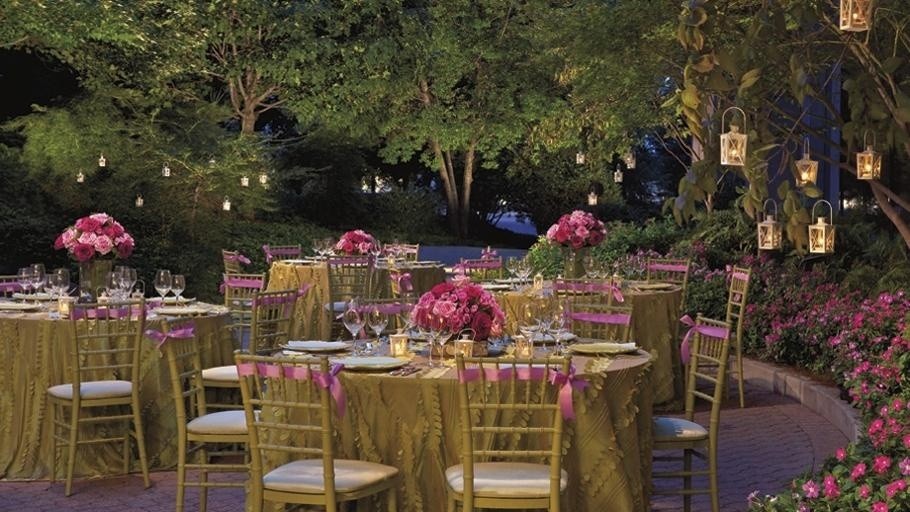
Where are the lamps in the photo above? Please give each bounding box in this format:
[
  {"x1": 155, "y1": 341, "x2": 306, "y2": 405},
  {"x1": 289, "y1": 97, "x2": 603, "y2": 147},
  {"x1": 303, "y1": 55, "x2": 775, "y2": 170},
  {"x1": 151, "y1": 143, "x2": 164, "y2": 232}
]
[
  {"x1": 839, "y1": 0, "x2": 872, "y2": 32},
  {"x1": 161, "y1": 161, "x2": 171, "y2": 177},
  {"x1": 98, "y1": 153, "x2": 106, "y2": 167},
  {"x1": 857, "y1": 128, "x2": 884, "y2": 180},
  {"x1": 758, "y1": 198, "x2": 782, "y2": 250},
  {"x1": 261, "y1": 174, "x2": 269, "y2": 185},
  {"x1": 76, "y1": 168, "x2": 85, "y2": 183},
  {"x1": 221, "y1": 195, "x2": 232, "y2": 210},
  {"x1": 135, "y1": 193, "x2": 142, "y2": 207},
  {"x1": 809, "y1": 201, "x2": 836, "y2": 253},
  {"x1": 719, "y1": 105, "x2": 750, "y2": 167},
  {"x1": 792, "y1": 136, "x2": 818, "y2": 190},
  {"x1": 241, "y1": 174, "x2": 248, "y2": 185}
]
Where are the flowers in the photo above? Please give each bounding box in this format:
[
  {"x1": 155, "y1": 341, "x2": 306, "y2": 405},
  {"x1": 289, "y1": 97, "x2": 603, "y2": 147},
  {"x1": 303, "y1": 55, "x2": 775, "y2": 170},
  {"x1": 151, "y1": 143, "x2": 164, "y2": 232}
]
[
  {"x1": 334, "y1": 229, "x2": 377, "y2": 252},
  {"x1": 545, "y1": 210, "x2": 607, "y2": 249},
  {"x1": 55, "y1": 212, "x2": 135, "y2": 263},
  {"x1": 409, "y1": 281, "x2": 507, "y2": 340}
]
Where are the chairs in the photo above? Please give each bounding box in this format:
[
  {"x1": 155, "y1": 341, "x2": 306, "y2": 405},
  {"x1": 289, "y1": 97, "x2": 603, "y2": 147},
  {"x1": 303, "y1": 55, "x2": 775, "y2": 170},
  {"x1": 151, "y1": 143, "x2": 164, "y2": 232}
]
[
  {"x1": 1, "y1": 274, "x2": 36, "y2": 299},
  {"x1": 647, "y1": 254, "x2": 692, "y2": 313},
  {"x1": 652, "y1": 314, "x2": 731, "y2": 511},
  {"x1": 46, "y1": 298, "x2": 151, "y2": 496},
  {"x1": 221, "y1": 248, "x2": 266, "y2": 352},
  {"x1": 445, "y1": 351, "x2": 574, "y2": 512},
  {"x1": 565, "y1": 304, "x2": 632, "y2": 345},
  {"x1": 160, "y1": 314, "x2": 259, "y2": 512},
  {"x1": 187, "y1": 288, "x2": 299, "y2": 418},
  {"x1": 266, "y1": 242, "x2": 302, "y2": 266},
  {"x1": 383, "y1": 243, "x2": 419, "y2": 263},
  {"x1": 680, "y1": 266, "x2": 752, "y2": 407},
  {"x1": 459, "y1": 256, "x2": 502, "y2": 283},
  {"x1": 553, "y1": 276, "x2": 612, "y2": 336},
  {"x1": 234, "y1": 353, "x2": 399, "y2": 510},
  {"x1": 328, "y1": 255, "x2": 376, "y2": 339}
]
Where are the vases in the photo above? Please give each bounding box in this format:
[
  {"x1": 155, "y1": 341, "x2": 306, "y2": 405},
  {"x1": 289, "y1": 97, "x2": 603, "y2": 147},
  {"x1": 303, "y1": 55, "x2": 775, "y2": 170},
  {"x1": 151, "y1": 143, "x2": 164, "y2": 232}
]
[
  {"x1": 348, "y1": 254, "x2": 369, "y2": 264},
  {"x1": 565, "y1": 244, "x2": 587, "y2": 281},
  {"x1": 431, "y1": 340, "x2": 488, "y2": 357},
  {"x1": 76, "y1": 257, "x2": 111, "y2": 301}
]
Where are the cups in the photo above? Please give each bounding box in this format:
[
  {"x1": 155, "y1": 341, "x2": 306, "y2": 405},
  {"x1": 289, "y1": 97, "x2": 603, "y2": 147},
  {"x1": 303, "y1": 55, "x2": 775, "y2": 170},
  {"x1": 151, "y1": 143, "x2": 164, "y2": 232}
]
[
  {"x1": 343, "y1": 298, "x2": 367, "y2": 358},
  {"x1": 545, "y1": 297, "x2": 568, "y2": 357},
  {"x1": 43, "y1": 274, "x2": 57, "y2": 304},
  {"x1": 52, "y1": 268, "x2": 71, "y2": 297},
  {"x1": 511, "y1": 332, "x2": 536, "y2": 360},
  {"x1": 57, "y1": 296, "x2": 79, "y2": 319},
  {"x1": 594, "y1": 261, "x2": 610, "y2": 280},
  {"x1": 430, "y1": 312, "x2": 453, "y2": 370},
  {"x1": 27, "y1": 263, "x2": 46, "y2": 294},
  {"x1": 519, "y1": 256, "x2": 533, "y2": 285},
  {"x1": 505, "y1": 256, "x2": 520, "y2": 285},
  {"x1": 170, "y1": 274, "x2": 185, "y2": 306},
  {"x1": 17, "y1": 268, "x2": 35, "y2": 294},
  {"x1": 389, "y1": 334, "x2": 409, "y2": 358},
  {"x1": 518, "y1": 300, "x2": 542, "y2": 357},
  {"x1": 582, "y1": 256, "x2": 602, "y2": 279},
  {"x1": 154, "y1": 269, "x2": 173, "y2": 305},
  {"x1": 366, "y1": 304, "x2": 389, "y2": 357},
  {"x1": 513, "y1": 260, "x2": 529, "y2": 285},
  {"x1": 531, "y1": 288, "x2": 554, "y2": 353},
  {"x1": 105, "y1": 267, "x2": 138, "y2": 304},
  {"x1": 416, "y1": 306, "x2": 443, "y2": 365}
]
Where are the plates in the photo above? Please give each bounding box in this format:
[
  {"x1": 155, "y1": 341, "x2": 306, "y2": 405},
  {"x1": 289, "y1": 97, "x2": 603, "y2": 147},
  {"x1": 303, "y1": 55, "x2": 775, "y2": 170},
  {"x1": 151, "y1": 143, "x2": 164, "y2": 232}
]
[
  {"x1": 556, "y1": 289, "x2": 600, "y2": 294},
  {"x1": 482, "y1": 285, "x2": 511, "y2": 290},
  {"x1": 565, "y1": 342, "x2": 644, "y2": 355},
  {"x1": 277, "y1": 342, "x2": 354, "y2": 351},
  {"x1": 493, "y1": 279, "x2": 533, "y2": 284},
  {"x1": 145, "y1": 298, "x2": 197, "y2": 303},
  {"x1": 0, "y1": 303, "x2": 43, "y2": 310},
  {"x1": 328, "y1": 356, "x2": 413, "y2": 372},
  {"x1": 12, "y1": 292, "x2": 68, "y2": 300},
  {"x1": 152, "y1": 307, "x2": 213, "y2": 315},
  {"x1": 636, "y1": 283, "x2": 673, "y2": 290}
]
